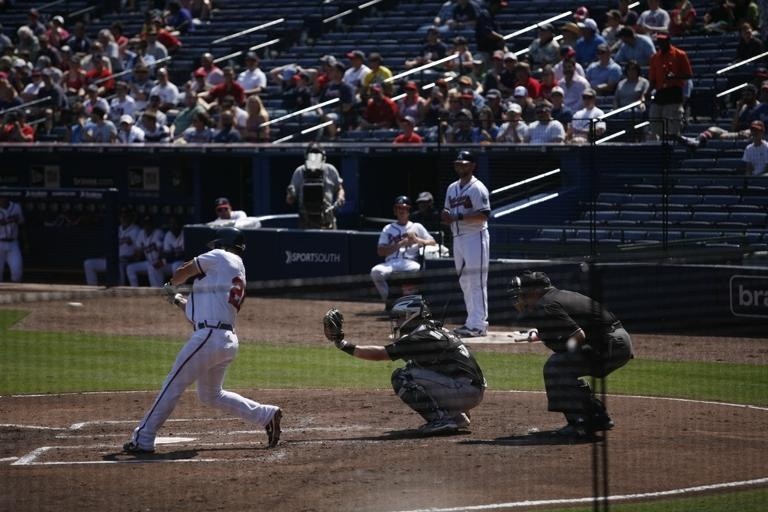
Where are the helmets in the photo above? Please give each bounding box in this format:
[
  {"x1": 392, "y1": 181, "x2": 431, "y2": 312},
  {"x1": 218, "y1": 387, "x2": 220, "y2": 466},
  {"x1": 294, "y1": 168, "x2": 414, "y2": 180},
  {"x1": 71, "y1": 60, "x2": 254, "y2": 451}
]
[
  {"x1": 391, "y1": 295, "x2": 430, "y2": 340},
  {"x1": 208, "y1": 228, "x2": 245, "y2": 250},
  {"x1": 506, "y1": 270, "x2": 550, "y2": 313}
]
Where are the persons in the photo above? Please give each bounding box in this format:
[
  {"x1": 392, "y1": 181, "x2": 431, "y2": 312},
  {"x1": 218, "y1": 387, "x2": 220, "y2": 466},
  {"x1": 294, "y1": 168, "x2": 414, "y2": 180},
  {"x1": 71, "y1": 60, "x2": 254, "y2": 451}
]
[
  {"x1": 121, "y1": 227, "x2": 283, "y2": 452},
  {"x1": 520, "y1": 270, "x2": 636, "y2": 438},
  {"x1": 1, "y1": 1, "x2": 768, "y2": 175},
  {"x1": 1, "y1": 191, "x2": 194, "y2": 288},
  {"x1": 205, "y1": 145, "x2": 492, "y2": 336},
  {"x1": 321, "y1": 293, "x2": 488, "y2": 432}
]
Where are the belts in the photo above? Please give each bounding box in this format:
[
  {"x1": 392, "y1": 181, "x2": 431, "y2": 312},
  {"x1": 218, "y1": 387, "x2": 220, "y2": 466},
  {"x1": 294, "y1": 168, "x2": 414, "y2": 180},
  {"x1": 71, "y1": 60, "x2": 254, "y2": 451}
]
[{"x1": 194, "y1": 324, "x2": 232, "y2": 333}]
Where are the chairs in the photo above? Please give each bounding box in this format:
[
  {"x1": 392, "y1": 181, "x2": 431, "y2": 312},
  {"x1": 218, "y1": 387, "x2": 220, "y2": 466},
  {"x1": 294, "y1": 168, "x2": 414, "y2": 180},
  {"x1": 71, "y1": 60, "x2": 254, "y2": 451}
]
[{"x1": 0, "y1": 0, "x2": 768, "y2": 270}]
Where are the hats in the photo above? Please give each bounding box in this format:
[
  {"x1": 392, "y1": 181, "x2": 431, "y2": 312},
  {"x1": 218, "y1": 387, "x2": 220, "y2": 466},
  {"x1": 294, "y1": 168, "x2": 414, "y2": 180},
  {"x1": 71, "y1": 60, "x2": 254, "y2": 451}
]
[
  {"x1": 455, "y1": 20, "x2": 670, "y2": 124},
  {"x1": 403, "y1": 117, "x2": 415, "y2": 125},
  {"x1": 751, "y1": 120, "x2": 765, "y2": 130},
  {"x1": 454, "y1": 151, "x2": 475, "y2": 165},
  {"x1": 214, "y1": 198, "x2": 230, "y2": 210},
  {"x1": 321, "y1": 51, "x2": 383, "y2": 70},
  {"x1": 403, "y1": 80, "x2": 414, "y2": 89},
  {"x1": 417, "y1": 192, "x2": 436, "y2": 203},
  {"x1": 395, "y1": 195, "x2": 411, "y2": 209}
]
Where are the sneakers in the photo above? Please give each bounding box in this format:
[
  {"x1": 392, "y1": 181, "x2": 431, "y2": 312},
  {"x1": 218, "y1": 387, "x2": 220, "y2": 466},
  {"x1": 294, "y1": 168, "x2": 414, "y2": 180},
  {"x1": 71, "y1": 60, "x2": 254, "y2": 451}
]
[
  {"x1": 122, "y1": 441, "x2": 155, "y2": 452},
  {"x1": 453, "y1": 327, "x2": 487, "y2": 337},
  {"x1": 556, "y1": 416, "x2": 615, "y2": 436},
  {"x1": 267, "y1": 409, "x2": 282, "y2": 448},
  {"x1": 420, "y1": 412, "x2": 470, "y2": 433},
  {"x1": 677, "y1": 135, "x2": 699, "y2": 150}
]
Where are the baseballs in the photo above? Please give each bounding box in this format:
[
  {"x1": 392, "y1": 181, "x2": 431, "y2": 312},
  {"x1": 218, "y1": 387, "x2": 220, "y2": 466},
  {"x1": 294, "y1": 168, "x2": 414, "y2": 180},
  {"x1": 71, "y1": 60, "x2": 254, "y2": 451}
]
[{"x1": 68, "y1": 300, "x2": 83, "y2": 310}]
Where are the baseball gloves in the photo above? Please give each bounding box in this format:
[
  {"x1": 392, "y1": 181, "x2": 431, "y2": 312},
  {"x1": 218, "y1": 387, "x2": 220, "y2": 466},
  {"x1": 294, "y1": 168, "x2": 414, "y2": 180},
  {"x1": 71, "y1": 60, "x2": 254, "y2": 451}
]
[{"x1": 323, "y1": 307, "x2": 344, "y2": 343}]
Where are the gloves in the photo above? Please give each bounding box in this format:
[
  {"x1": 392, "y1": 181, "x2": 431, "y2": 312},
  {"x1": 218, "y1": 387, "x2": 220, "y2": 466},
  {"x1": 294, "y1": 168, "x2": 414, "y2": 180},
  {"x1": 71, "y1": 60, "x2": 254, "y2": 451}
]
[{"x1": 162, "y1": 281, "x2": 183, "y2": 306}]
